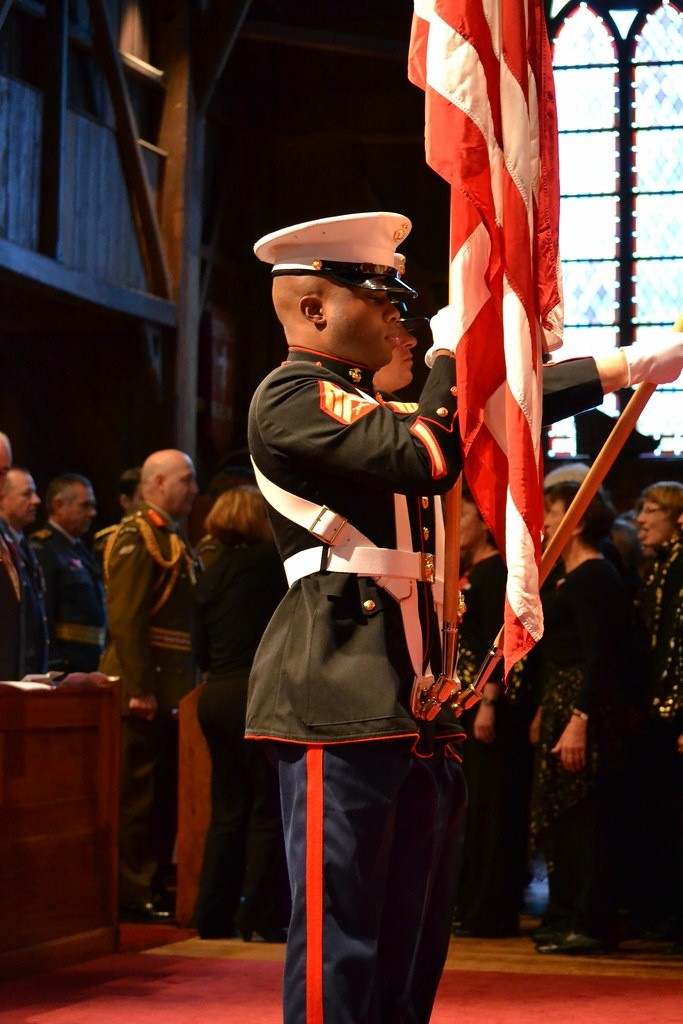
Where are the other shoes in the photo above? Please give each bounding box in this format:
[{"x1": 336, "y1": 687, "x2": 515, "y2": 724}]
[{"x1": 453, "y1": 917, "x2": 518, "y2": 939}]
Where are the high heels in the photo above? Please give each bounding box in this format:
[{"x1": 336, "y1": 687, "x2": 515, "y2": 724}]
[{"x1": 237, "y1": 898, "x2": 288, "y2": 943}]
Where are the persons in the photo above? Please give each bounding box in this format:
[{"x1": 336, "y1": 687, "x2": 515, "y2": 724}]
[
  {"x1": 25, "y1": 472, "x2": 105, "y2": 683},
  {"x1": 246, "y1": 211, "x2": 683, "y2": 1024},
  {"x1": 370, "y1": 249, "x2": 417, "y2": 391},
  {"x1": 94, "y1": 441, "x2": 245, "y2": 921},
  {"x1": 460, "y1": 460, "x2": 683, "y2": 958},
  {"x1": 193, "y1": 484, "x2": 287, "y2": 941},
  {"x1": 0, "y1": 465, "x2": 53, "y2": 683},
  {"x1": 0, "y1": 430, "x2": 25, "y2": 689}
]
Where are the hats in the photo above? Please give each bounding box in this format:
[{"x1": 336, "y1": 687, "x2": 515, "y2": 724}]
[
  {"x1": 254, "y1": 212, "x2": 418, "y2": 298},
  {"x1": 393, "y1": 253, "x2": 429, "y2": 331}
]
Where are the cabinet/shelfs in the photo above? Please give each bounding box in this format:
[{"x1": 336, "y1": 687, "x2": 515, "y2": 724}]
[{"x1": 0, "y1": 673, "x2": 123, "y2": 981}]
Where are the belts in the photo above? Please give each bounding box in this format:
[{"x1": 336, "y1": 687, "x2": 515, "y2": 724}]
[{"x1": 283, "y1": 546, "x2": 434, "y2": 588}]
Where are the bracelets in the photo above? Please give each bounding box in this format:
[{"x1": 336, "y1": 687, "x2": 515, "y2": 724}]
[
  {"x1": 571, "y1": 711, "x2": 589, "y2": 721},
  {"x1": 483, "y1": 695, "x2": 496, "y2": 707}
]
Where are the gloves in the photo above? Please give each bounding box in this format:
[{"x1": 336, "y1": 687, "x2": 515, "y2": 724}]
[
  {"x1": 425, "y1": 304, "x2": 456, "y2": 368},
  {"x1": 620, "y1": 328, "x2": 683, "y2": 388}
]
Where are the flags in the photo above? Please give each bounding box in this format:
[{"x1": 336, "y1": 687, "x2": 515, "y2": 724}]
[{"x1": 401, "y1": 1, "x2": 567, "y2": 693}]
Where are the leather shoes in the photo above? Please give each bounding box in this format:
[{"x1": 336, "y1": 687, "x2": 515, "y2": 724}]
[
  {"x1": 118, "y1": 902, "x2": 175, "y2": 924},
  {"x1": 531, "y1": 931, "x2": 602, "y2": 955}
]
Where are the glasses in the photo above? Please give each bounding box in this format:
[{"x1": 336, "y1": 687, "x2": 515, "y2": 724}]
[{"x1": 641, "y1": 508, "x2": 661, "y2": 514}]
